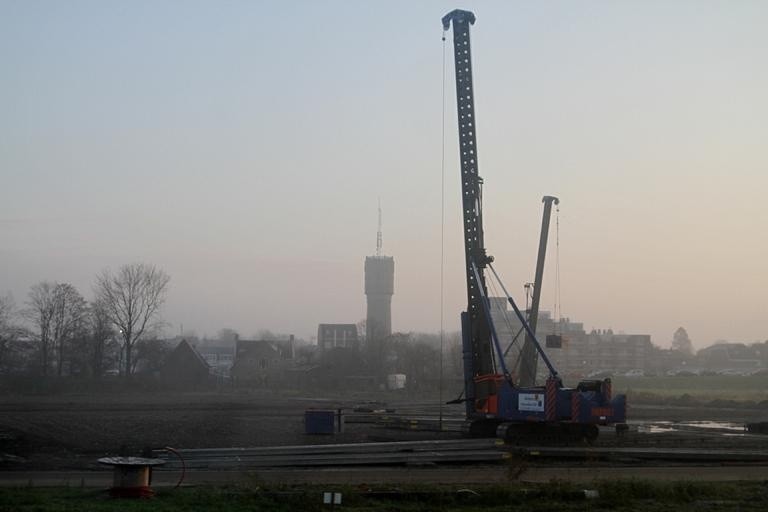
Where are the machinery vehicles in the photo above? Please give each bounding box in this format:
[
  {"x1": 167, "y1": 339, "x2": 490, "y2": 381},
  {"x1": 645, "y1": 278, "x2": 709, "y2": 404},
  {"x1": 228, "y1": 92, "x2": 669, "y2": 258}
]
[{"x1": 441, "y1": 7, "x2": 629, "y2": 448}]
